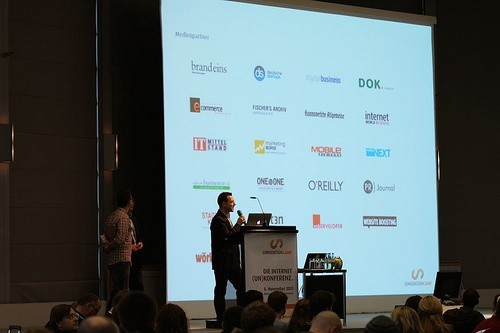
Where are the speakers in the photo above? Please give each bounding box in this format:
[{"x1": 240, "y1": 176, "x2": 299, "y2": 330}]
[{"x1": 434, "y1": 271, "x2": 465, "y2": 304}]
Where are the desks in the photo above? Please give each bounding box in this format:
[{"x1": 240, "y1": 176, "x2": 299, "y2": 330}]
[{"x1": 298, "y1": 269, "x2": 347, "y2": 327}]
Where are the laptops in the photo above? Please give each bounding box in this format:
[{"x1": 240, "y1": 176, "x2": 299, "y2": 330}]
[
  {"x1": 304, "y1": 253, "x2": 326, "y2": 269},
  {"x1": 246, "y1": 213, "x2": 272, "y2": 226}
]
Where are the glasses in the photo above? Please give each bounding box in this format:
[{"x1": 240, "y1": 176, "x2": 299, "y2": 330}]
[
  {"x1": 63, "y1": 313, "x2": 80, "y2": 319},
  {"x1": 132, "y1": 201, "x2": 135, "y2": 203},
  {"x1": 92, "y1": 304, "x2": 99, "y2": 313}
]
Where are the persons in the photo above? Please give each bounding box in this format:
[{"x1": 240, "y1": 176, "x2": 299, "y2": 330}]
[
  {"x1": 40, "y1": 287, "x2": 500, "y2": 333},
  {"x1": 210, "y1": 192, "x2": 246, "y2": 315},
  {"x1": 128, "y1": 197, "x2": 144, "y2": 293},
  {"x1": 101, "y1": 192, "x2": 132, "y2": 314}
]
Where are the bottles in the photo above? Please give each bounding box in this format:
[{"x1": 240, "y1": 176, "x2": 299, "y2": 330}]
[{"x1": 309, "y1": 253, "x2": 336, "y2": 270}]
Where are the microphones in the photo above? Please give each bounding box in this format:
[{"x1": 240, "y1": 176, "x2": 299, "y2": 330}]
[
  {"x1": 251, "y1": 197, "x2": 267, "y2": 226},
  {"x1": 237, "y1": 210, "x2": 247, "y2": 226}
]
